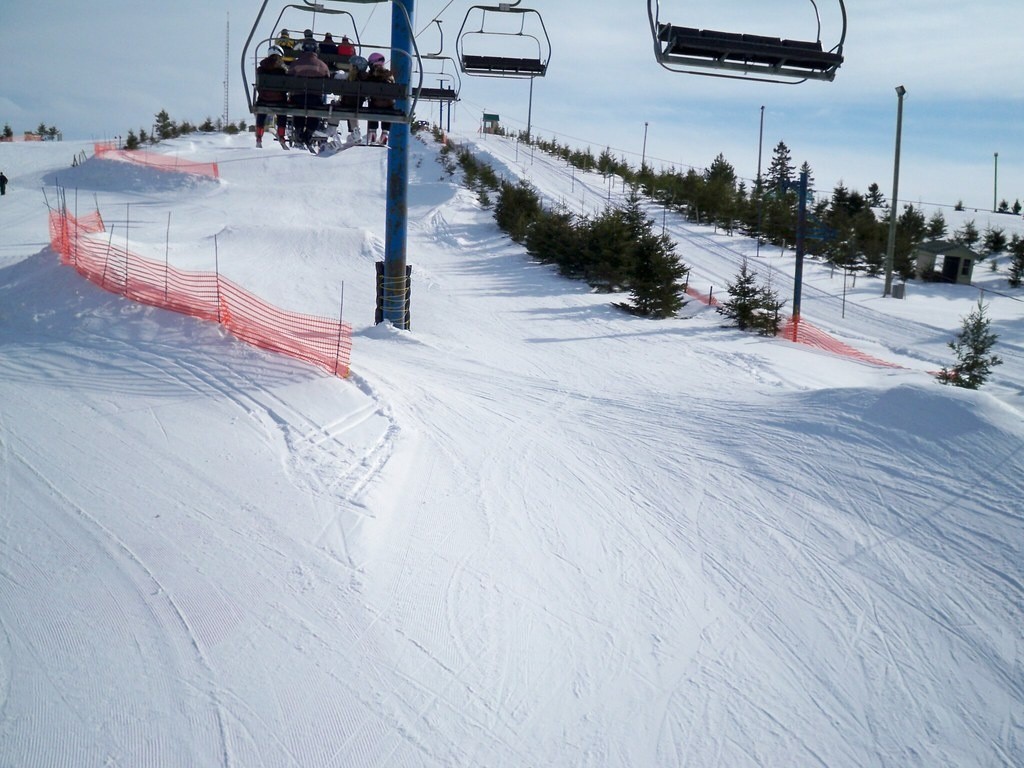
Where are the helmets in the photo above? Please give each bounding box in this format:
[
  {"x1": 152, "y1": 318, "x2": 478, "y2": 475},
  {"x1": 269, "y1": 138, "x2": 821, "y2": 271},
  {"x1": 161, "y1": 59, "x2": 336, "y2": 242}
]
[
  {"x1": 369, "y1": 52, "x2": 385, "y2": 68},
  {"x1": 281, "y1": 29, "x2": 289, "y2": 36},
  {"x1": 302, "y1": 37, "x2": 317, "y2": 52},
  {"x1": 349, "y1": 56, "x2": 367, "y2": 71},
  {"x1": 268, "y1": 45, "x2": 284, "y2": 58}
]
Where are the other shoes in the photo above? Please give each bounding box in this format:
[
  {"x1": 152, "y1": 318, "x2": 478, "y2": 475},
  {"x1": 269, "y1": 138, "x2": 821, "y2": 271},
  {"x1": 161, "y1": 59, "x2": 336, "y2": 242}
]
[
  {"x1": 347, "y1": 130, "x2": 362, "y2": 143},
  {"x1": 367, "y1": 129, "x2": 377, "y2": 143},
  {"x1": 302, "y1": 129, "x2": 312, "y2": 143},
  {"x1": 294, "y1": 128, "x2": 302, "y2": 142},
  {"x1": 256, "y1": 136, "x2": 261, "y2": 142},
  {"x1": 279, "y1": 137, "x2": 286, "y2": 144},
  {"x1": 381, "y1": 130, "x2": 388, "y2": 144}
]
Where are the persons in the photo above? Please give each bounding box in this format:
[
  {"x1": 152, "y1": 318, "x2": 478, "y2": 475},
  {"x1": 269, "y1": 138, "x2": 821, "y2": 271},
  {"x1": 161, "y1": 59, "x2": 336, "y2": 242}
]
[
  {"x1": 0, "y1": 172, "x2": 8, "y2": 195},
  {"x1": 256, "y1": 28, "x2": 396, "y2": 151}
]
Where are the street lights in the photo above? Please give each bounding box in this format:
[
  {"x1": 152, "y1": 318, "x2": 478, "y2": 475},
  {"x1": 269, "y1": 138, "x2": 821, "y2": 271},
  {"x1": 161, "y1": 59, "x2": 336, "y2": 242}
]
[
  {"x1": 756, "y1": 105, "x2": 767, "y2": 196},
  {"x1": 640, "y1": 122, "x2": 649, "y2": 173},
  {"x1": 993, "y1": 152, "x2": 999, "y2": 212},
  {"x1": 883, "y1": 85, "x2": 908, "y2": 299}
]
